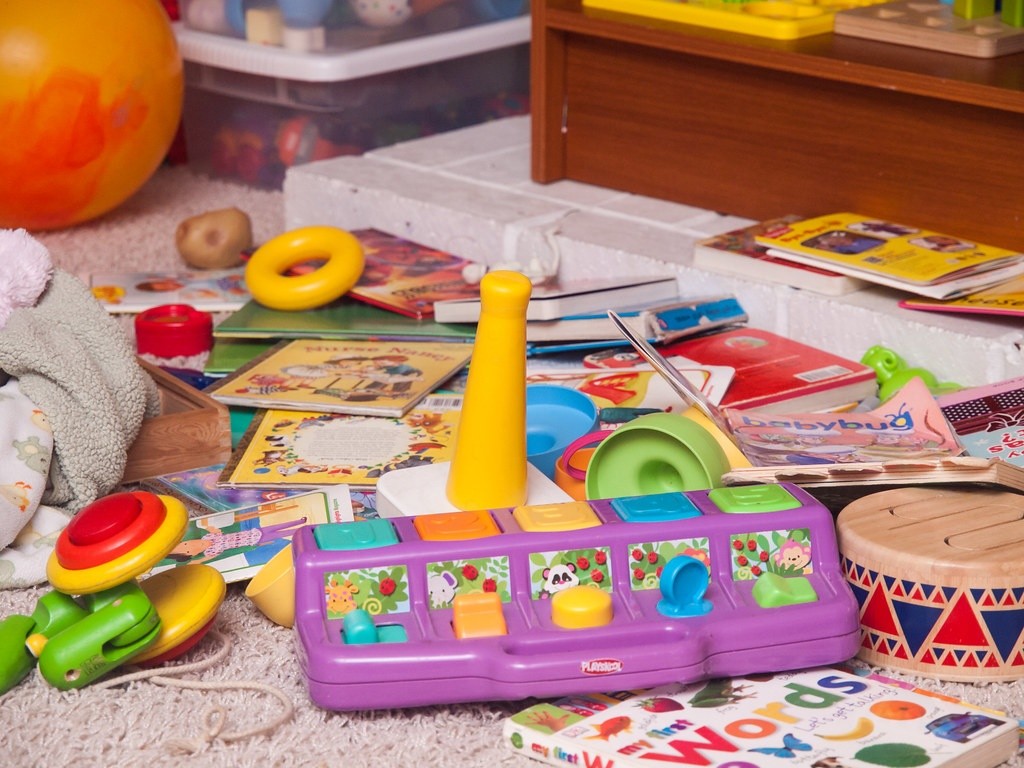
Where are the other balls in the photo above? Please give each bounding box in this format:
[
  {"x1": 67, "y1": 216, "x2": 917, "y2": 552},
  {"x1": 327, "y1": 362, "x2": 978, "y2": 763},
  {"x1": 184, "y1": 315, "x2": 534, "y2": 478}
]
[{"x1": 0, "y1": 2, "x2": 187, "y2": 233}]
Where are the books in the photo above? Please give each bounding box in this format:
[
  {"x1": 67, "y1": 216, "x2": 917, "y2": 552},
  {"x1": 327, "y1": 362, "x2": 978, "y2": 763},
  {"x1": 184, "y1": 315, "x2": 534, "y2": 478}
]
[{"x1": 82, "y1": 208, "x2": 1024, "y2": 768}]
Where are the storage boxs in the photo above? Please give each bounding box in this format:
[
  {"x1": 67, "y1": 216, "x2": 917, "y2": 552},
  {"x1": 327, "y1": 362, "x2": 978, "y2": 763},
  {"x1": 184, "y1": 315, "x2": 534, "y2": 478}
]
[{"x1": 169, "y1": 11, "x2": 531, "y2": 198}]
